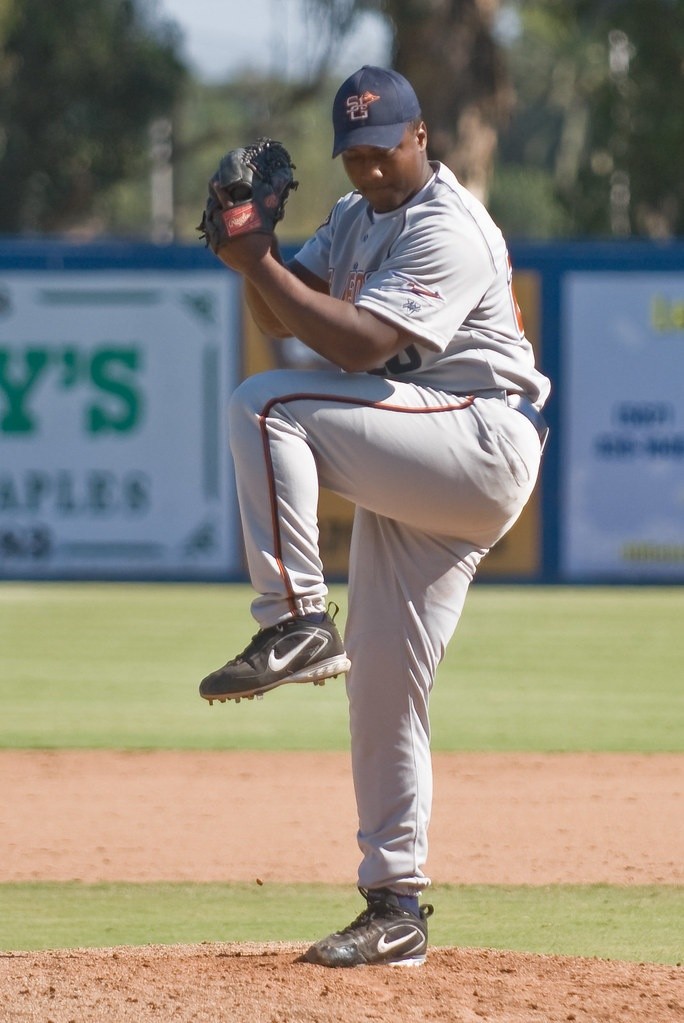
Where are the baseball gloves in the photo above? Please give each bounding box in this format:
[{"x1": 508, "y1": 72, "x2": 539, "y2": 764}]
[{"x1": 201, "y1": 136, "x2": 293, "y2": 256}]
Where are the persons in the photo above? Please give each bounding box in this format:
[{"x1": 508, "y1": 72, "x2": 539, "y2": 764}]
[{"x1": 199, "y1": 65, "x2": 553, "y2": 965}]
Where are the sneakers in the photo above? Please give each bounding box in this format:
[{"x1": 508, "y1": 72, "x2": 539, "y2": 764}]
[
  {"x1": 305, "y1": 886, "x2": 434, "y2": 967},
  {"x1": 200, "y1": 601, "x2": 352, "y2": 706}
]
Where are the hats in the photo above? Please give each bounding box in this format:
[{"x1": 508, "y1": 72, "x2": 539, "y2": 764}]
[{"x1": 331, "y1": 64, "x2": 421, "y2": 159}]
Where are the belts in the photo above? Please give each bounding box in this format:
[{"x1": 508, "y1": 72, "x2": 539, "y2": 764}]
[{"x1": 507, "y1": 393, "x2": 548, "y2": 444}]
